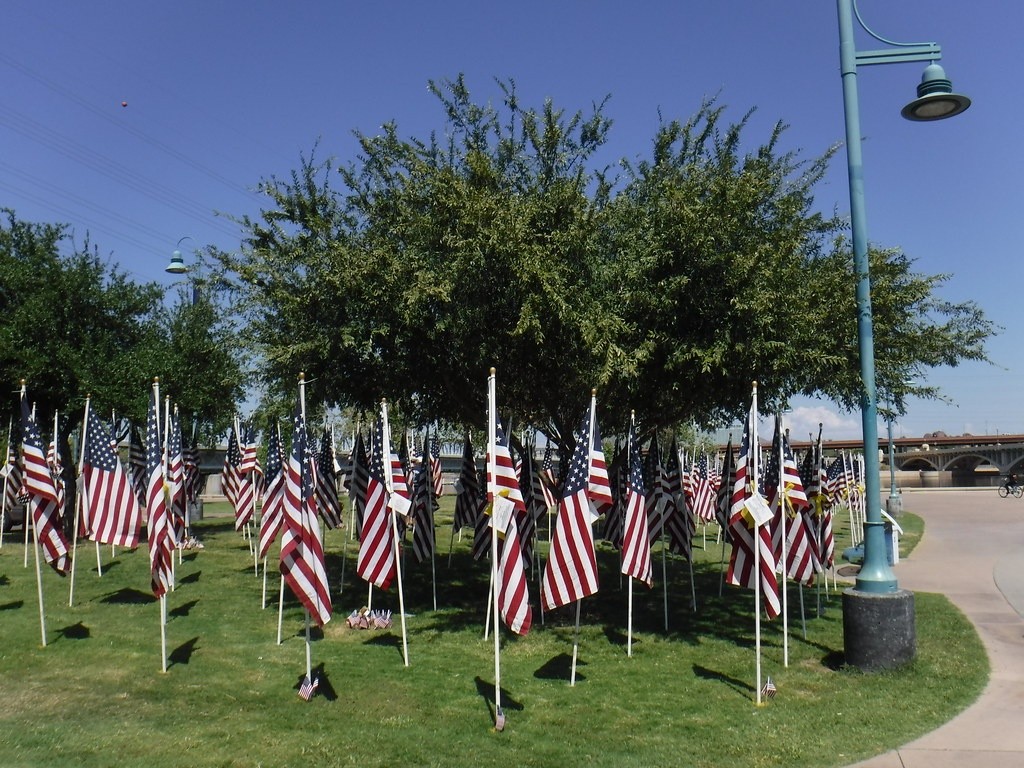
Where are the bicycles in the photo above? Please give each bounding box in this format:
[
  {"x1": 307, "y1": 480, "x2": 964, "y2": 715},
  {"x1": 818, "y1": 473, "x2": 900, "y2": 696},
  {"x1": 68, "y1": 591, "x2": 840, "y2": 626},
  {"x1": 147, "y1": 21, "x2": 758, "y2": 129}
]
[{"x1": 998, "y1": 478, "x2": 1023, "y2": 498}]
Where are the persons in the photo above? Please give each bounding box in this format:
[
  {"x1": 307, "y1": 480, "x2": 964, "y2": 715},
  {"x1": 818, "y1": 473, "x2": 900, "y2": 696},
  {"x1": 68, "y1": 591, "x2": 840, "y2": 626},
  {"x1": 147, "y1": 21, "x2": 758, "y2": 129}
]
[{"x1": 1005, "y1": 469, "x2": 1018, "y2": 494}]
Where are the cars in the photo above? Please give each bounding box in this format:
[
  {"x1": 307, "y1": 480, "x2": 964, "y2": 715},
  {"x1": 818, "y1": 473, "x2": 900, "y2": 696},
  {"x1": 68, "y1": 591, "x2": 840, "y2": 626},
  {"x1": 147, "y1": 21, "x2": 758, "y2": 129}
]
[{"x1": 0, "y1": 478, "x2": 32, "y2": 533}]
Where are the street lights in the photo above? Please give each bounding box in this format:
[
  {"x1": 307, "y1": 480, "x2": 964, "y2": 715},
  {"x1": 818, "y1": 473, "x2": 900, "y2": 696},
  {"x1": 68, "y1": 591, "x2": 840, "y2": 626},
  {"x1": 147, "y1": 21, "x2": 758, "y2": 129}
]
[
  {"x1": 887, "y1": 377, "x2": 916, "y2": 497},
  {"x1": 165, "y1": 235, "x2": 203, "y2": 438},
  {"x1": 842, "y1": 43, "x2": 972, "y2": 584}
]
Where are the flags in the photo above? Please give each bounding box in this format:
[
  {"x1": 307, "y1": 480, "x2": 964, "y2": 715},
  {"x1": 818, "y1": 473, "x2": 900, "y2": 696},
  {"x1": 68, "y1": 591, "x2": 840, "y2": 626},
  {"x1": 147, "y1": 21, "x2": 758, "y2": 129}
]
[
  {"x1": 452, "y1": 405, "x2": 868, "y2": 620},
  {"x1": 260, "y1": 417, "x2": 285, "y2": 558},
  {"x1": 540, "y1": 402, "x2": 613, "y2": 611},
  {"x1": 343, "y1": 421, "x2": 442, "y2": 561},
  {"x1": 145, "y1": 390, "x2": 176, "y2": 595},
  {"x1": 81, "y1": 407, "x2": 142, "y2": 549},
  {"x1": 128, "y1": 416, "x2": 146, "y2": 506},
  {"x1": 21, "y1": 395, "x2": 70, "y2": 577},
  {"x1": 235, "y1": 421, "x2": 265, "y2": 531},
  {"x1": 621, "y1": 424, "x2": 654, "y2": 590},
  {"x1": 6, "y1": 421, "x2": 24, "y2": 512},
  {"x1": 278, "y1": 395, "x2": 333, "y2": 627},
  {"x1": 16, "y1": 421, "x2": 39, "y2": 503},
  {"x1": 357, "y1": 403, "x2": 409, "y2": 592},
  {"x1": 308, "y1": 422, "x2": 343, "y2": 530},
  {"x1": 46, "y1": 428, "x2": 66, "y2": 518},
  {"x1": 486, "y1": 393, "x2": 533, "y2": 636},
  {"x1": 164, "y1": 407, "x2": 206, "y2": 549},
  {"x1": 221, "y1": 423, "x2": 242, "y2": 508}
]
